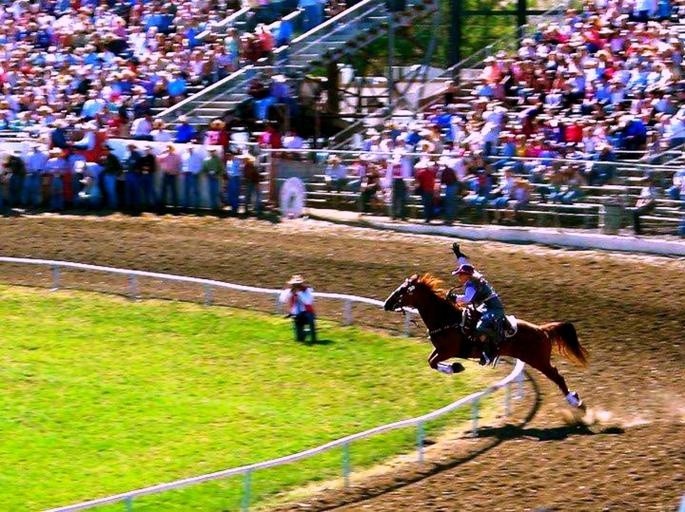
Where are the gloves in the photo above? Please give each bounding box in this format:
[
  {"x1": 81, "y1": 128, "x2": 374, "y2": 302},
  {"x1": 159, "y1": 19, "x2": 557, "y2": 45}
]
[
  {"x1": 451, "y1": 243, "x2": 465, "y2": 260},
  {"x1": 446, "y1": 293, "x2": 458, "y2": 304}
]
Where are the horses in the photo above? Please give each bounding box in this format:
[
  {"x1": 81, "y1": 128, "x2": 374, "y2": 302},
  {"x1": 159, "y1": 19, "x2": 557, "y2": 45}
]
[{"x1": 383, "y1": 271, "x2": 592, "y2": 413}]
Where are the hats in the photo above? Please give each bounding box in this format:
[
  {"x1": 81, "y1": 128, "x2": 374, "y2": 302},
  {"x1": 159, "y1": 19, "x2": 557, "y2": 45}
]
[
  {"x1": 451, "y1": 264, "x2": 474, "y2": 276},
  {"x1": 286, "y1": 275, "x2": 305, "y2": 285}
]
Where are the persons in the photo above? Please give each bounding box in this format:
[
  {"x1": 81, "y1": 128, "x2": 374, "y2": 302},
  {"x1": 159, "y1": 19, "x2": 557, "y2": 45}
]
[
  {"x1": 1, "y1": 1, "x2": 684, "y2": 244},
  {"x1": 280, "y1": 272, "x2": 316, "y2": 344},
  {"x1": 440, "y1": 241, "x2": 505, "y2": 367}
]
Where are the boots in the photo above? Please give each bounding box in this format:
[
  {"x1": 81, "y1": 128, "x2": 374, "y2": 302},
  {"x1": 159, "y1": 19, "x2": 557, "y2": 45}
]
[{"x1": 479, "y1": 337, "x2": 497, "y2": 366}]
